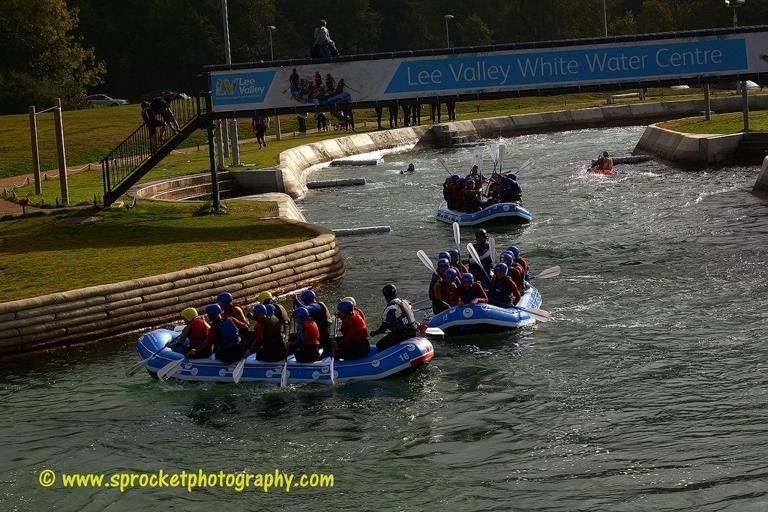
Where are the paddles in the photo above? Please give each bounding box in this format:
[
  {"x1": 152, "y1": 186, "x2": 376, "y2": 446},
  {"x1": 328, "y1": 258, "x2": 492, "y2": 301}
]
[
  {"x1": 232, "y1": 338, "x2": 256, "y2": 384},
  {"x1": 490, "y1": 298, "x2": 549, "y2": 323},
  {"x1": 437, "y1": 158, "x2": 452, "y2": 176},
  {"x1": 473, "y1": 146, "x2": 485, "y2": 200},
  {"x1": 515, "y1": 157, "x2": 536, "y2": 174},
  {"x1": 453, "y1": 222, "x2": 461, "y2": 263},
  {"x1": 329, "y1": 313, "x2": 337, "y2": 382},
  {"x1": 526, "y1": 265, "x2": 560, "y2": 280},
  {"x1": 385, "y1": 327, "x2": 445, "y2": 335},
  {"x1": 489, "y1": 238, "x2": 496, "y2": 268},
  {"x1": 417, "y1": 249, "x2": 443, "y2": 280},
  {"x1": 175, "y1": 325, "x2": 187, "y2": 334},
  {"x1": 280, "y1": 354, "x2": 288, "y2": 387},
  {"x1": 467, "y1": 243, "x2": 490, "y2": 281},
  {"x1": 125, "y1": 333, "x2": 182, "y2": 377},
  {"x1": 485, "y1": 143, "x2": 505, "y2": 193},
  {"x1": 157, "y1": 352, "x2": 191, "y2": 382}
]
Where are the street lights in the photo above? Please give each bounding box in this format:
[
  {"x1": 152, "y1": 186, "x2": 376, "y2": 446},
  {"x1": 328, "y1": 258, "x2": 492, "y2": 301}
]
[
  {"x1": 724, "y1": 0, "x2": 746, "y2": 27},
  {"x1": 443, "y1": 13, "x2": 455, "y2": 49},
  {"x1": 265, "y1": 24, "x2": 277, "y2": 61}
]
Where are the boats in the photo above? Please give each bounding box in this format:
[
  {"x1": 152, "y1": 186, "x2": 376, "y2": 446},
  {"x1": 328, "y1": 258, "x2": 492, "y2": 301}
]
[
  {"x1": 134, "y1": 316, "x2": 441, "y2": 390},
  {"x1": 435, "y1": 196, "x2": 532, "y2": 227},
  {"x1": 585, "y1": 165, "x2": 617, "y2": 177},
  {"x1": 422, "y1": 279, "x2": 543, "y2": 337}
]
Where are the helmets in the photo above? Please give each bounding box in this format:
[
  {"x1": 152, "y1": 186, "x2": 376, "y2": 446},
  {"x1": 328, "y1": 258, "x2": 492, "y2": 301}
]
[
  {"x1": 382, "y1": 283, "x2": 397, "y2": 296},
  {"x1": 180, "y1": 307, "x2": 197, "y2": 322},
  {"x1": 298, "y1": 288, "x2": 317, "y2": 300},
  {"x1": 494, "y1": 245, "x2": 522, "y2": 272},
  {"x1": 253, "y1": 291, "x2": 278, "y2": 316},
  {"x1": 216, "y1": 291, "x2": 233, "y2": 304},
  {"x1": 439, "y1": 248, "x2": 476, "y2": 283},
  {"x1": 293, "y1": 307, "x2": 310, "y2": 319},
  {"x1": 449, "y1": 173, "x2": 476, "y2": 189},
  {"x1": 206, "y1": 304, "x2": 223, "y2": 317},
  {"x1": 337, "y1": 296, "x2": 357, "y2": 314}
]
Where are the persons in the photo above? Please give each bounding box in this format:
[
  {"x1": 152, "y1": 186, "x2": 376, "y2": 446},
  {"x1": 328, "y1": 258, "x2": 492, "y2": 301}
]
[
  {"x1": 311, "y1": 20, "x2": 328, "y2": 43},
  {"x1": 165, "y1": 164, "x2": 529, "y2": 365},
  {"x1": 141, "y1": 89, "x2": 458, "y2": 152},
  {"x1": 288, "y1": 68, "x2": 349, "y2": 100},
  {"x1": 310, "y1": 28, "x2": 340, "y2": 57},
  {"x1": 590, "y1": 150, "x2": 613, "y2": 171}
]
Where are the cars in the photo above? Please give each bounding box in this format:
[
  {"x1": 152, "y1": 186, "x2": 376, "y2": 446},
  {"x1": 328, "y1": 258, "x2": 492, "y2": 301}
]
[
  {"x1": 669, "y1": 85, "x2": 691, "y2": 89},
  {"x1": 84, "y1": 93, "x2": 131, "y2": 106},
  {"x1": 141, "y1": 90, "x2": 191, "y2": 104},
  {"x1": 735, "y1": 79, "x2": 761, "y2": 90}
]
